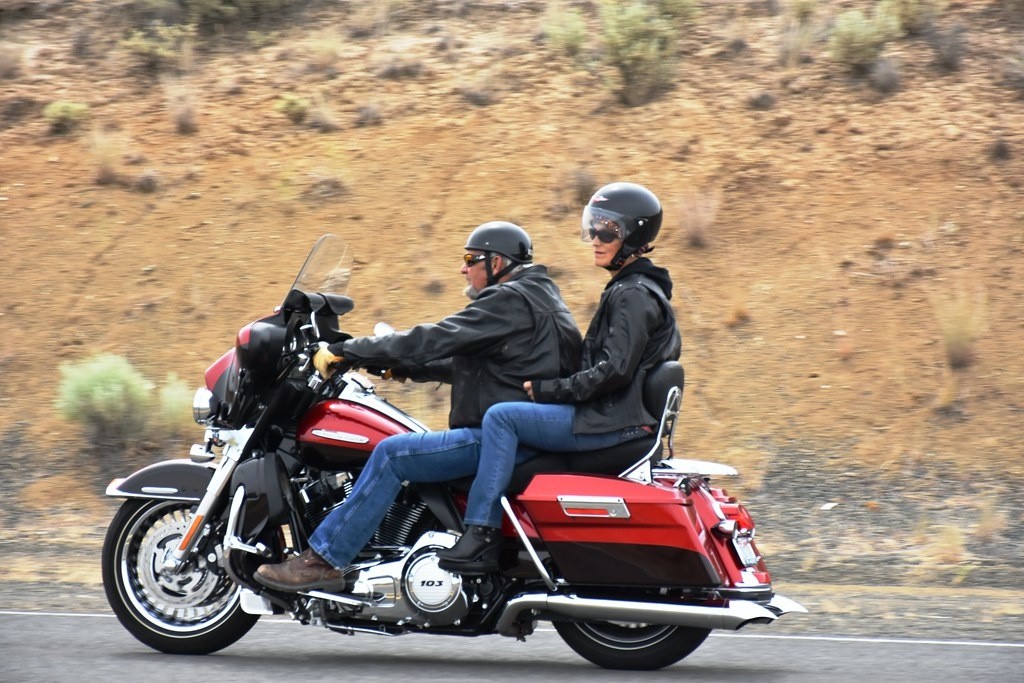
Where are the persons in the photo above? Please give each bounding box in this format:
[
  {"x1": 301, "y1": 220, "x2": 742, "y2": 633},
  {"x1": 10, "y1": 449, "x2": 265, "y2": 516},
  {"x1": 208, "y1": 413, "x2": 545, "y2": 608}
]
[
  {"x1": 437, "y1": 183, "x2": 681, "y2": 565},
  {"x1": 252, "y1": 221, "x2": 584, "y2": 593}
]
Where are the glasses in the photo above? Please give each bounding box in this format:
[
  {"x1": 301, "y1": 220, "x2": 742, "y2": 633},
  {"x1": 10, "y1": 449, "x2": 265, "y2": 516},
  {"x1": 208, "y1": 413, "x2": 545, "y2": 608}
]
[
  {"x1": 588, "y1": 227, "x2": 619, "y2": 243},
  {"x1": 463, "y1": 254, "x2": 494, "y2": 267}
]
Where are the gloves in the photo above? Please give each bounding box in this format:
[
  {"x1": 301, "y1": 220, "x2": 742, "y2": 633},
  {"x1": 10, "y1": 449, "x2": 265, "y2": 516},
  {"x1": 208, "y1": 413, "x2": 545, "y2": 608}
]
[
  {"x1": 312, "y1": 342, "x2": 343, "y2": 379},
  {"x1": 383, "y1": 365, "x2": 408, "y2": 383}
]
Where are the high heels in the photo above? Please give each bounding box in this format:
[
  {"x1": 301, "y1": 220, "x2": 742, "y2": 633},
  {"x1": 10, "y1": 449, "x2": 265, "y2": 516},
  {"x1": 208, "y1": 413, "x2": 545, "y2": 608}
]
[{"x1": 434, "y1": 525, "x2": 501, "y2": 563}]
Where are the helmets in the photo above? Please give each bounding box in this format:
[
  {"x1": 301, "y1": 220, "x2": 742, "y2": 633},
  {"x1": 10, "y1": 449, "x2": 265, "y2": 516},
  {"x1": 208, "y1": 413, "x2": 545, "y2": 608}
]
[
  {"x1": 464, "y1": 221, "x2": 534, "y2": 264},
  {"x1": 581, "y1": 182, "x2": 663, "y2": 247}
]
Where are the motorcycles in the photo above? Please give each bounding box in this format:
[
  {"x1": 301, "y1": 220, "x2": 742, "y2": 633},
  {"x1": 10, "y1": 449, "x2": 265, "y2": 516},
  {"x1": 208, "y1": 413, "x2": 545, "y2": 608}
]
[{"x1": 98, "y1": 233, "x2": 810, "y2": 672}]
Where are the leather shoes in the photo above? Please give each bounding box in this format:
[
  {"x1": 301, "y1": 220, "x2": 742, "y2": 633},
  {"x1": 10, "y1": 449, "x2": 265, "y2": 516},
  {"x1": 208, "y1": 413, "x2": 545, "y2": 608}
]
[{"x1": 253, "y1": 550, "x2": 346, "y2": 594}]
